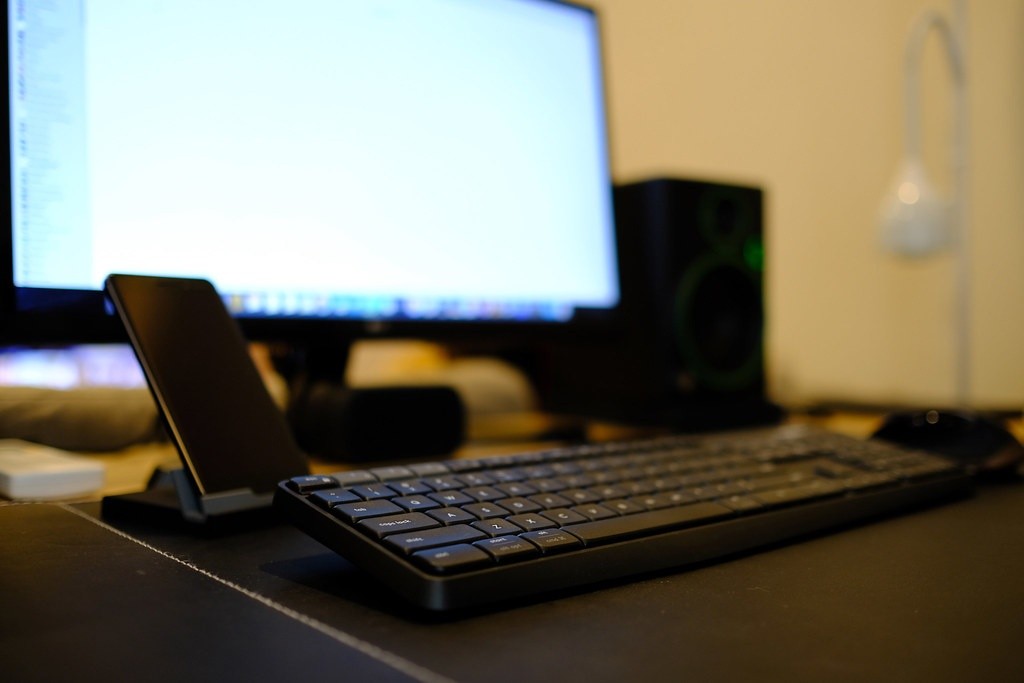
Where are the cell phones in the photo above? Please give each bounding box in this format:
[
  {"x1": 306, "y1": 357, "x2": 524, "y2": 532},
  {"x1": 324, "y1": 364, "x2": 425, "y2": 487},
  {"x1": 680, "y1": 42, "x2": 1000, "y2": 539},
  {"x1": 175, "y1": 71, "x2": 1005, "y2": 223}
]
[{"x1": 106, "y1": 274, "x2": 311, "y2": 497}]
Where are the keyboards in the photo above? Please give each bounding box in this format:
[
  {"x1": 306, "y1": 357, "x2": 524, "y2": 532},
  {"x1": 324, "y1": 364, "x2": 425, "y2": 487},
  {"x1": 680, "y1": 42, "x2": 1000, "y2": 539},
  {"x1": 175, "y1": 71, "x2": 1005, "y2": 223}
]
[{"x1": 272, "y1": 425, "x2": 977, "y2": 628}]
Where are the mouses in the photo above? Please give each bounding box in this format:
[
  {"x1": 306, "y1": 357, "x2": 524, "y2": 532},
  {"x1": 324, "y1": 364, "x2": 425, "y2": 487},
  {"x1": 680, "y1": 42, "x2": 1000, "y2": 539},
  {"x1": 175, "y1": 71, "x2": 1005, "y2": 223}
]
[{"x1": 869, "y1": 411, "x2": 1024, "y2": 488}]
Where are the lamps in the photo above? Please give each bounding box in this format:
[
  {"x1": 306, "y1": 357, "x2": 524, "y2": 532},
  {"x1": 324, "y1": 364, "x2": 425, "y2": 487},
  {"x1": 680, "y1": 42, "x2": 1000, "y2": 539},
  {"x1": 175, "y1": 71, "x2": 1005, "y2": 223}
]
[{"x1": 873, "y1": 15, "x2": 981, "y2": 407}]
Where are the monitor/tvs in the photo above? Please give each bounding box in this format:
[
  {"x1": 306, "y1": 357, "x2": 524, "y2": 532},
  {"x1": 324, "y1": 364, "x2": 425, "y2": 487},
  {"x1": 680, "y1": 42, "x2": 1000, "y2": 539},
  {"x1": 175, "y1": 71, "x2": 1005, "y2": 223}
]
[{"x1": 0, "y1": 0, "x2": 622, "y2": 468}]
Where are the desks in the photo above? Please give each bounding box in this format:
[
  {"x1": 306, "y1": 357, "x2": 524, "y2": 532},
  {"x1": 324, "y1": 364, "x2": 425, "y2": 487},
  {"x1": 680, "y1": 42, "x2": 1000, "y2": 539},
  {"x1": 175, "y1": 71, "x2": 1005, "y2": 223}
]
[{"x1": 0, "y1": 404, "x2": 1024, "y2": 683}]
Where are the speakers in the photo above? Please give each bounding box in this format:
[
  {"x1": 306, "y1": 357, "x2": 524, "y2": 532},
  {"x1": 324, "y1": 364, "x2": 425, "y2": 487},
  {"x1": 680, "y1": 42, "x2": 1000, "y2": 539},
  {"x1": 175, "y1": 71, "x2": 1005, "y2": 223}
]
[{"x1": 522, "y1": 179, "x2": 767, "y2": 434}]
